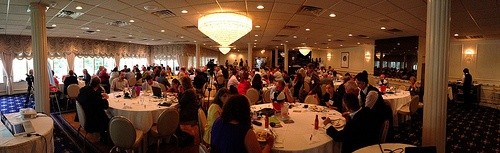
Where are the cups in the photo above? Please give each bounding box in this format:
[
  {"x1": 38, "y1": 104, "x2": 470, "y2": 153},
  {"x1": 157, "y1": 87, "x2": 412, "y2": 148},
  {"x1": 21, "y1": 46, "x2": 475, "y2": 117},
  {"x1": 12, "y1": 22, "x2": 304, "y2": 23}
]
[{"x1": 140, "y1": 96, "x2": 144, "y2": 105}]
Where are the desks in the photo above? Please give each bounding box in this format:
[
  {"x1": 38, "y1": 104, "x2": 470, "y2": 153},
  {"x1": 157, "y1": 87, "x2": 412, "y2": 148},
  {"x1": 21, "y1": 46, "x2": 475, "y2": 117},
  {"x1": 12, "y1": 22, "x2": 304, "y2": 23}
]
[
  {"x1": 447, "y1": 81, "x2": 481, "y2": 103},
  {"x1": 0, "y1": 111, "x2": 54, "y2": 153}
]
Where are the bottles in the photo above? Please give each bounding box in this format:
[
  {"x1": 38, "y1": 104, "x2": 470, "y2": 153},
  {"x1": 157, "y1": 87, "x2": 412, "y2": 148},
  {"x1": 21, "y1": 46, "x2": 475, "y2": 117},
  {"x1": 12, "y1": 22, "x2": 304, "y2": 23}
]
[
  {"x1": 315, "y1": 115, "x2": 318, "y2": 129},
  {"x1": 265, "y1": 113, "x2": 269, "y2": 128},
  {"x1": 281, "y1": 104, "x2": 288, "y2": 121}
]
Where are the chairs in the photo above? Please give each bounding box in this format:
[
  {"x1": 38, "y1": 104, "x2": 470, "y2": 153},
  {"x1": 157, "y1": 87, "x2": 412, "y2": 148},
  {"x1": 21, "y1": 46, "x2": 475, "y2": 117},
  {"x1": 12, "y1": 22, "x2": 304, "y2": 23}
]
[
  {"x1": 65, "y1": 83, "x2": 83, "y2": 111},
  {"x1": 197, "y1": 109, "x2": 208, "y2": 143},
  {"x1": 303, "y1": 95, "x2": 319, "y2": 105},
  {"x1": 202, "y1": 84, "x2": 216, "y2": 106},
  {"x1": 263, "y1": 89, "x2": 272, "y2": 103},
  {"x1": 149, "y1": 108, "x2": 181, "y2": 151},
  {"x1": 397, "y1": 95, "x2": 419, "y2": 123},
  {"x1": 246, "y1": 88, "x2": 259, "y2": 104},
  {"x1": 199, "y1": 143, "x2": 208, "y2": 153},
  {"x1": 73, "y1": 101, "x2": 107, "y2": 150},
  {"x1": 108, "y1": 115, "x2": 136, "y2": 153}
]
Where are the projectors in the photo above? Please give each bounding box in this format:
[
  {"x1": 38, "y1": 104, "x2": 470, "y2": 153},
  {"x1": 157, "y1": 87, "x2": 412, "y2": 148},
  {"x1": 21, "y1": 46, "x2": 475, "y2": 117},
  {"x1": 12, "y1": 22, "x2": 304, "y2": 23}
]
[{"x1": 0, "y1": 111, "x2": 35, "y2": 137}]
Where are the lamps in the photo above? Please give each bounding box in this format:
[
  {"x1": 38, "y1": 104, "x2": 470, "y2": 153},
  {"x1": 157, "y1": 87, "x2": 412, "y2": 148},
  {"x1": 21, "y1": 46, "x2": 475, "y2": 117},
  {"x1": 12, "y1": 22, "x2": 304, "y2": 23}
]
[
  {"x1": 298, "y1": 48, "x2": 313, "y2": 56},
  {"x1": 194, "y1": 13, "x2": 252, "y2": 49},
  {"x1": 218, "y1": 47, "x2": 231, "y2": 55},
  {"x1": 463, "y1": 48, "x2": 474, "y2": 64}
]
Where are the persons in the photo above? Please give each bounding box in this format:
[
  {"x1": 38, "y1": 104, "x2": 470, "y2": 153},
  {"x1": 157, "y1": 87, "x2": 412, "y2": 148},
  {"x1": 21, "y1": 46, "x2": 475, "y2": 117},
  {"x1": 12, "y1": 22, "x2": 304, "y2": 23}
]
[
  {"x1": 25, "y1": 70, "x2": 34, "y2": 104},
  {"x1": 354, "y1": 70, "x2": 386, "y2": 132},
  {"x1": 109, "y1": 70, "x2": 129, "y2": 92},
  {"x1": 133, "y1": 59, "x2": 359, "y2": 112},
  {"x1": 121, "y1": 65, "x2": 129, "y2": 72},
  {"x1": 97, "y1": 66, "x2": 108, "y2": 81},
  {"x1": 378, "y1": 73, "x2": 388, "y2": 86},
  {"x1": 323, "y1": 93, "x2": 384, "y2": 153},
  {"x1": 79, "y1": 69, "x2": 91, "y2": 86},
  {"x1": 125, "y1": 68, "x2": 132, "y2": 79},
  {"x1": 407, "y1": 76, "x2": 423, "y2": 97},
  {"x1": 110, "y1": 67, "x2": 119, "y2": 77},
  {"x1": 461, "y1": 68, "x2": 473, "y2": 111},
  {"x1": 210, "y1": 94, "x2": 274, "y2": 153},
  {"x1": 73, "y1": 76, "x2": 115, "y2": 147},
  {"x1": 374, "y1": 65, "x2": 417, "y2": 80},
  {"x1": 49, "y1": 70, "x2": 64, "y2": 103},
  {"x1": 89, "y1": 74, "x2": 105, "y2": 93},
  {"x1": 64, "y1": 70, "x2": 78, "y2": 95}
]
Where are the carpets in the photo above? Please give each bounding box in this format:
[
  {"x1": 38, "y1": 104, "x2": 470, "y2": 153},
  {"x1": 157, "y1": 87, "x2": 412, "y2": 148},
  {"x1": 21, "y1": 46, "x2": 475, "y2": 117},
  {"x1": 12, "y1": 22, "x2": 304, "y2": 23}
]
[
  {"x1": 50, "y1": 109, "x2": 114, "y2": 152},
  {"x1": 0, "y1": 92, "x2": 81, "y2": 153}
]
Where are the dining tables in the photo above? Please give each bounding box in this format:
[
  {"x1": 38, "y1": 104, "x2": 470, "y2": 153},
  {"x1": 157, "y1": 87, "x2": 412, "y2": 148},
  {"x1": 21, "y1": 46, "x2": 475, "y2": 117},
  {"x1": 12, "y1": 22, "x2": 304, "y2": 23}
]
[
  {"x1": 253, "y1": 83, "x2": 278, "y2": 99},
  {"x1": 250, "y1": 102, "x2": 346, "y2": 151},
  {"x1": 374, "y1": 85, "x2": 410, "y2": 122},
  {"x1": 102, "y1": 90, "x2": 179, "y2": 152},
  {"x1": 55, "y1": 76, "x2": 85, "y2": 94},
  {"x1": 310, "y1": 80, "x2": 346, "y2": 91}
]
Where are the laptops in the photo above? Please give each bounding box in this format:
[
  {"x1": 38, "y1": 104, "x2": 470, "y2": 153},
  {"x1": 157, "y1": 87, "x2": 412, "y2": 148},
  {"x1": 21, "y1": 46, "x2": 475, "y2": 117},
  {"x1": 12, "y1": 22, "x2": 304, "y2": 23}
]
[{"x1": 405, "y1": 146, "x2": 438, "y2": 153}]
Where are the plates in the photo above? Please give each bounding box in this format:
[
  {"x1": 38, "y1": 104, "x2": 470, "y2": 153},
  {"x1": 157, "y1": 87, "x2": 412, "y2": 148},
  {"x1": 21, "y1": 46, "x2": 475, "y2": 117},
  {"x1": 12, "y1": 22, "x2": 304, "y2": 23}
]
[
  {"x1": 253, "y1": 129, "x2": 275, "y2": 142},
  {"x1": 315, "y1": 106, "x2": 324, "y2": 112},
  {"x1": 331, "y1": 120, "x2": 344, "y2": 128}
]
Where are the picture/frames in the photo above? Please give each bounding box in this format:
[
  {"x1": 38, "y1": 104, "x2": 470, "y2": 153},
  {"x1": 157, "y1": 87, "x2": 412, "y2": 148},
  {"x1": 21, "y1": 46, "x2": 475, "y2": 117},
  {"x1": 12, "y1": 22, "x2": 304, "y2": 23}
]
[{"x1": 340, "y1": 52, "x2": 349, "y2": 68}]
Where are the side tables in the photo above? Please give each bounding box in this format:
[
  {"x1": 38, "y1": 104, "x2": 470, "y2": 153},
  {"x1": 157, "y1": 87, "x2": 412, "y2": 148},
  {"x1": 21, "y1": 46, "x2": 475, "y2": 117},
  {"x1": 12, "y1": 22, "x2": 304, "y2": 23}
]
[{"x1": 164, "y1": 74, "x2": 182, "y2": 88}]
